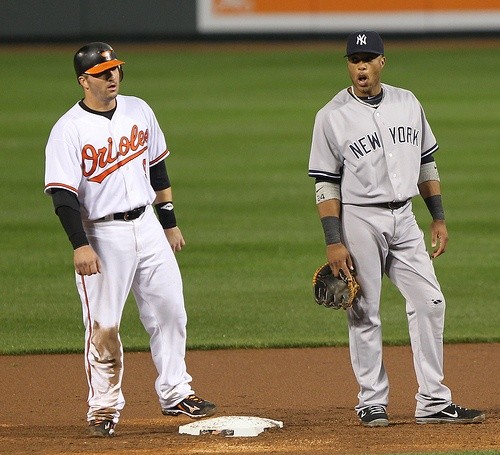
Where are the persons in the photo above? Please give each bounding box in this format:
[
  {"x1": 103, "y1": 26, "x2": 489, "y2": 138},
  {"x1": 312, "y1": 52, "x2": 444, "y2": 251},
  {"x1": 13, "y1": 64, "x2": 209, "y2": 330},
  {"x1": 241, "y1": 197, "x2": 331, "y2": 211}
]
[
  {"x1": 307, "y1": 32, "x2": 486, "y2": 427},
  {"x1": 45, "y1": 41, "x2": 218, "y2": 437}
]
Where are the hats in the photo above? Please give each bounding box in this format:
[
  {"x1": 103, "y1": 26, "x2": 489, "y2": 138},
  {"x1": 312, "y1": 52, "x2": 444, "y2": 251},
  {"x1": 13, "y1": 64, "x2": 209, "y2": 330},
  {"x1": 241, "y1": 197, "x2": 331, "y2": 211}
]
[{"x1": 343, "y1": 31, "x2": 384, "y2": 56}]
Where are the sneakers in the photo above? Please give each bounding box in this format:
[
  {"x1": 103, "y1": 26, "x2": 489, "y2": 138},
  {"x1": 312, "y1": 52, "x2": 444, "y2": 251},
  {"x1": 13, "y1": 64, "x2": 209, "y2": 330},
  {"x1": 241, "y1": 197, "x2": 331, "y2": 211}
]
[
  {"x1": 415, "y1": 404, "x2": 485, "y2": 424},
  {"x1": 359, "y1": 406, "x2": 390, "y2": 427},
  {"x1": 88, "y1": 420, "x2": 116, "y2": 437},
  {"x1": 162, "y1": 395, "x2": 216, "y2": 419}
]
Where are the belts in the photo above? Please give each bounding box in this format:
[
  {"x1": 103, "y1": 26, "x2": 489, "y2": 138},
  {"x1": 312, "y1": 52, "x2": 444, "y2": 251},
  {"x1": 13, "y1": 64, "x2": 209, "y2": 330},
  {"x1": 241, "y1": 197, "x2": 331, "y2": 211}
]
[
  {"x1": 341, "y1": 197, "x2": 411, "y2": 210},
  {"x1": 95, "y1": 206, "x2": 146, "y2": 221}
]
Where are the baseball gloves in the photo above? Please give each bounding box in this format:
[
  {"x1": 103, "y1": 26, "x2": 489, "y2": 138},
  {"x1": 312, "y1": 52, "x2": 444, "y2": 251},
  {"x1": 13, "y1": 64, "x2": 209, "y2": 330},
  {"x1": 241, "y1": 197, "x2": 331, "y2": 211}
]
[{"x1": 312, "y1": 260, "x2": 362, "y2": 310}]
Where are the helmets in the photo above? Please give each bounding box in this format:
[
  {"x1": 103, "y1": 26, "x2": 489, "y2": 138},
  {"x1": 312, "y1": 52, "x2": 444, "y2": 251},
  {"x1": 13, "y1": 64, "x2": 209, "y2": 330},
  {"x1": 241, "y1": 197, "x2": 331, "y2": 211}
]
[{"x1": 74, "y1": 43, "x2": 125, "y2": 74}]
